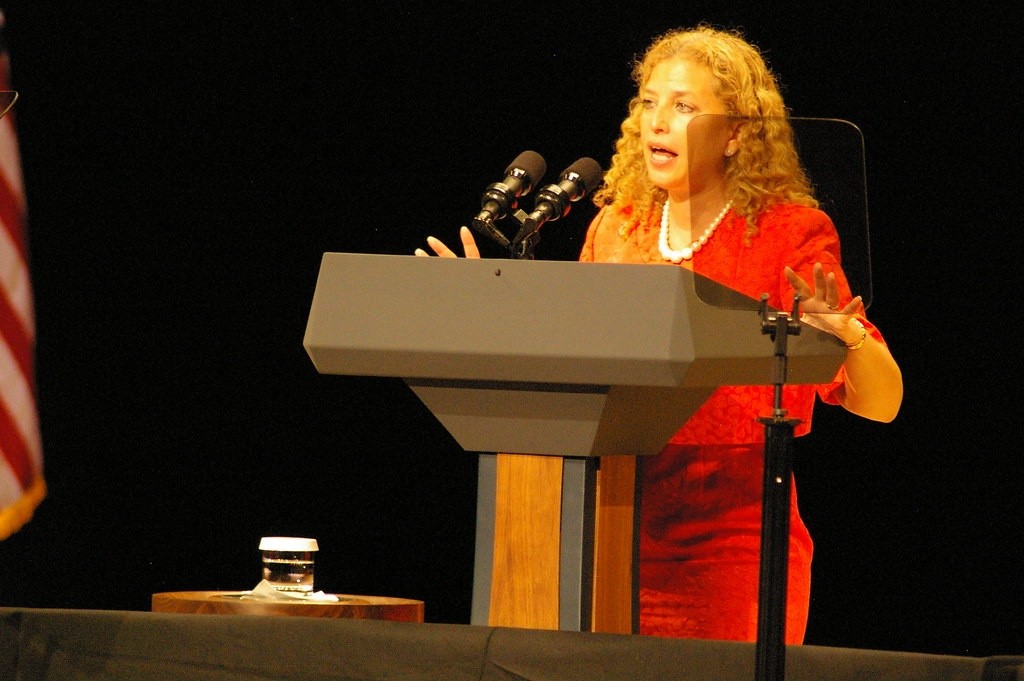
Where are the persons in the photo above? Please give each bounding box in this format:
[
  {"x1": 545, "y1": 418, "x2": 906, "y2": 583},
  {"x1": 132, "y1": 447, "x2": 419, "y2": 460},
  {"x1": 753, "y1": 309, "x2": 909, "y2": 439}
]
[{"x1": 415, "y1": 25, "x2": 905, "y2": 645}]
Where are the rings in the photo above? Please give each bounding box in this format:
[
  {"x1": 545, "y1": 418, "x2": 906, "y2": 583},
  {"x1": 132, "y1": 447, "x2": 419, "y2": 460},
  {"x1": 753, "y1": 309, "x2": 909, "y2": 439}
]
[{"x1": 826, "y1": 303, "x2": 839, "y2": 309}]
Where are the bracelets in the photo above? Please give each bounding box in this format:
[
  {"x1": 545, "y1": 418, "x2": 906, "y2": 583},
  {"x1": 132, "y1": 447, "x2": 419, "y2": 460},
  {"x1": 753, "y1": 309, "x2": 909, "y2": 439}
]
[{"x1": 848, "y1": 317, "x2": 866, "y2": 350}]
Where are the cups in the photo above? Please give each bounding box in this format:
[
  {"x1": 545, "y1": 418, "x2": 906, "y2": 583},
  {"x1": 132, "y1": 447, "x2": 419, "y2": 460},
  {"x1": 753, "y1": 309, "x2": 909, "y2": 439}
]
[{"x1": 258, "y1": 537, "x2": 318, "y2": 594}]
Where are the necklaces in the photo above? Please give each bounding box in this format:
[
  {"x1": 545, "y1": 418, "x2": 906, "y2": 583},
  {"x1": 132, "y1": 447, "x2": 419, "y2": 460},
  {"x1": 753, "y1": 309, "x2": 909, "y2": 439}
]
[{"x1": 658, "y1": 197, "x2": 733, "y2": 264}]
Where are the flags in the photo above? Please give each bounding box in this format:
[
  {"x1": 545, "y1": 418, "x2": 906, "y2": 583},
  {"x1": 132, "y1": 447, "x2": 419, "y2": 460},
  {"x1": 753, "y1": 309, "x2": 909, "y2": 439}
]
[{"x1": 0, "y1": 51, "x2": 46, "y2": 539}]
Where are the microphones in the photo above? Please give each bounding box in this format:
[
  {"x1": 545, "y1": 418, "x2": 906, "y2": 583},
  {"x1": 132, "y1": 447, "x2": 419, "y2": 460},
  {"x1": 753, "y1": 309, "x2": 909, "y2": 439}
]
[
  {"x1": 525, "y1": 157, "x2": 603, "y2": 232},
  {"x1": 472, "y1": 151, "x2": 547, "y2": 231}
]
